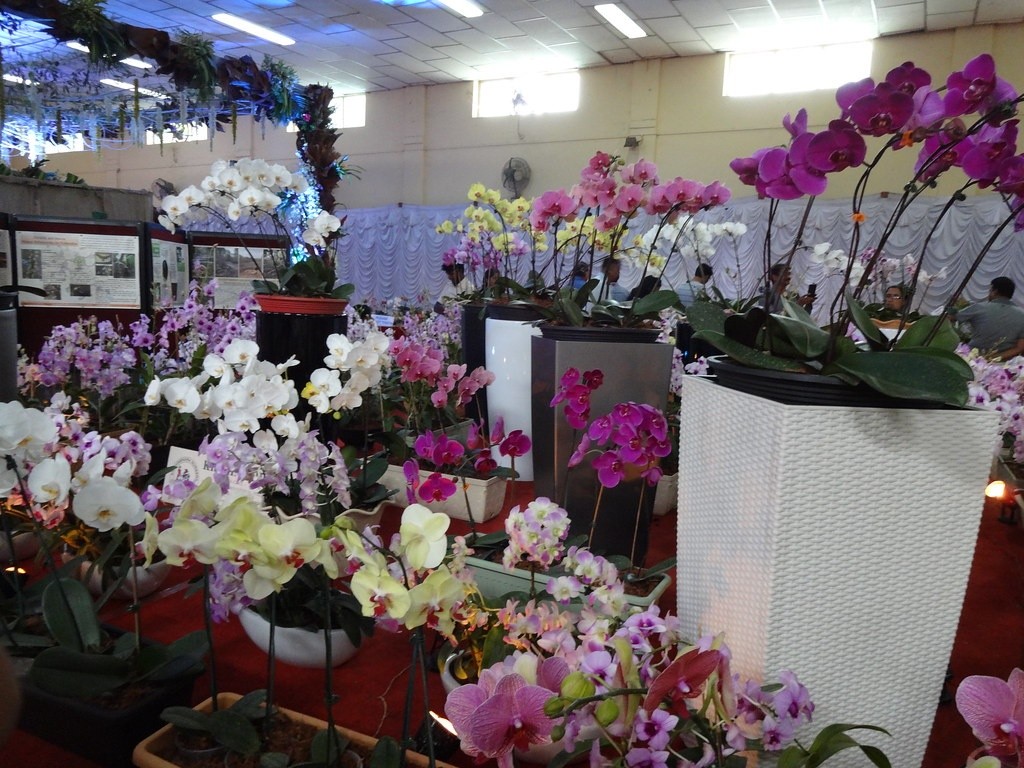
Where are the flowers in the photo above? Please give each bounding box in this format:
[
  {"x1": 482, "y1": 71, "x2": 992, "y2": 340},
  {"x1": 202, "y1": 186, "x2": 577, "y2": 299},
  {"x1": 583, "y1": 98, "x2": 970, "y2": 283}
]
[{"x1": 0, "y1": 1, "x2": 1024, "y2": 767}]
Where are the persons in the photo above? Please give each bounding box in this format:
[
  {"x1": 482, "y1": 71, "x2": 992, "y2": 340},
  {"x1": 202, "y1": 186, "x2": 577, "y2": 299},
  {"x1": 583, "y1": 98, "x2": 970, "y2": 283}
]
[
  {"x1": 564, "y1": 261, "x2": 590, "y2": 290},
  {"x1": 943, "y1": 276, "x2": 1024, "y2": 363},
  {"x1": 675, "y1": 262, "x2": 714, "y2": 309},
  {"x1": 625, "y1": 275, "x2": 662, "y2": 302},
  {"x1": 580, "y1": 256, "x2": 622, "y2": 318},
  {"x1": 883, "y1": 284, "x2": 912, "y2": 314},
  {"x1": 466, "y1": 268, "x2": 505, "y2": 303},
  {"x1": 433, "y1": 259, "x2": 477, "y2": 316},
  {"x1": 757, "y1": 264, "x2": 818, "y2": 318}
]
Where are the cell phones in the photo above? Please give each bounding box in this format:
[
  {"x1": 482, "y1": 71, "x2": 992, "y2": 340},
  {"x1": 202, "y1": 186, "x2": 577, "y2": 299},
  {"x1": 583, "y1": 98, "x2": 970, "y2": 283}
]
[{"x1": 808, "y1": 284, "x2": 817, "y2": 298}]
